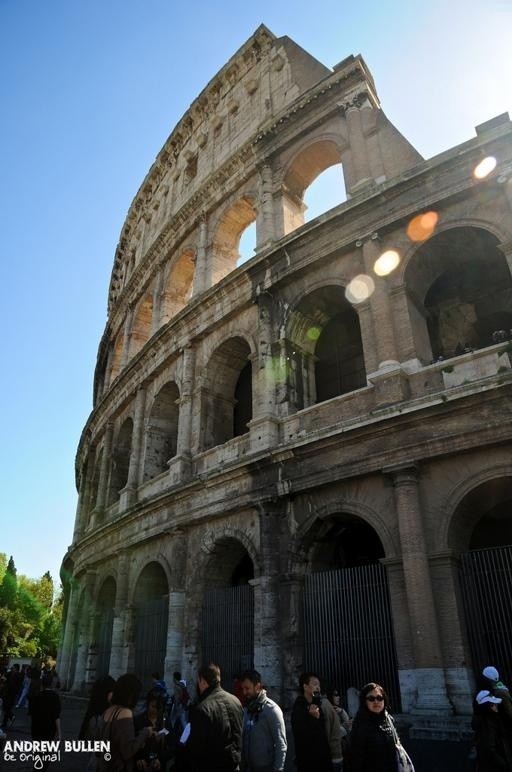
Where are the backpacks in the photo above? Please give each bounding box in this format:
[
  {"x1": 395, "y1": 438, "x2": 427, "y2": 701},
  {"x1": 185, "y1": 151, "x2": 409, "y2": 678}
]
[{"x1": 175, "y1": 684, "x2": 194, "y2": 709}]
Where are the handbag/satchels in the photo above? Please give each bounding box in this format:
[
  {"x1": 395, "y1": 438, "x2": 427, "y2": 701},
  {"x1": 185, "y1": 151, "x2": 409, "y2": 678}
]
[{"x1": 395, "y1": 742, "x2": 416, "y2": 771}]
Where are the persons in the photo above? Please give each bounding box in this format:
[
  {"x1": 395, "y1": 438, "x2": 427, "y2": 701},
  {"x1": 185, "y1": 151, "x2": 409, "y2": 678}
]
[{"x1": 468, "y1": 666, "x2": 512, "y2": 772}]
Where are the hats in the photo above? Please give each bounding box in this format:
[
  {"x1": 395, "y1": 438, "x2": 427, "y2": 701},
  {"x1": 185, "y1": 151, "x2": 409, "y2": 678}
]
[{"x1": 475, "y1": 666, "x2": 502, "y2": 704}]
